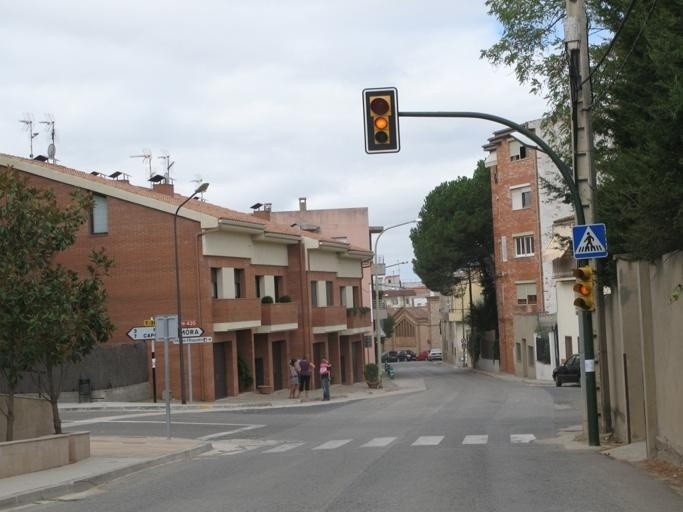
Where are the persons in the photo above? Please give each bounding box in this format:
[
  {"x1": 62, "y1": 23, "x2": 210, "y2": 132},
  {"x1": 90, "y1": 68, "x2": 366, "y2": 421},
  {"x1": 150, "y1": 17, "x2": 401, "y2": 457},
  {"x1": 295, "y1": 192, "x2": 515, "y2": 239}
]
[
  {"x1": 320, "y1": 359, "x2": 331, "y2": 381},
  {"x1": 287, "y1": 358, "x2": 299, "y2": 400},
  {"x1": 294, "y1": 355, "x2": 316, "y2": 399},
  {"x1": 319, "y1": 357, "x2": 332, "y2": 401}
]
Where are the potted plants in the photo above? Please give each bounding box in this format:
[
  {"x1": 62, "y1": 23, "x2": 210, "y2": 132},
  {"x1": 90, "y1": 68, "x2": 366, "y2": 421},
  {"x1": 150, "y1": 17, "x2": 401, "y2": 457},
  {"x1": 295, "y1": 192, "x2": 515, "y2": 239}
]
[{"x1": 364, "y1": 364, "x2": 379, "y2": 388}]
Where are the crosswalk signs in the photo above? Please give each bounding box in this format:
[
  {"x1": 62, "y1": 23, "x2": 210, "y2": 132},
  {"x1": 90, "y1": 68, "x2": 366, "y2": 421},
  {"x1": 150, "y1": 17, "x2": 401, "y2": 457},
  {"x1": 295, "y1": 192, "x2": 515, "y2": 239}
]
[{"x1": 572, "y1": 221, "x2": 610, "y2": 260}]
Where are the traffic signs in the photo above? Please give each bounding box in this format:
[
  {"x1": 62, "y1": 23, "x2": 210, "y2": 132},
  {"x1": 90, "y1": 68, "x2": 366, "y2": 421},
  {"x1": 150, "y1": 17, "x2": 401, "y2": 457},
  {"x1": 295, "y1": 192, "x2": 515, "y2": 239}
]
[{"x1": 125, "y1": 314, "x2": 212, "y2": 346}]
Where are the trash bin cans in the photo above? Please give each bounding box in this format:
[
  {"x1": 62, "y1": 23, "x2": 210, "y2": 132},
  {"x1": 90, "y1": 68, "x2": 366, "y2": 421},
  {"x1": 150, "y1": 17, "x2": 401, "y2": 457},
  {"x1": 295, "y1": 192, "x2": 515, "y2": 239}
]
[{"x1": 79, "y1": 379, "x2": 91, "y2": 403}]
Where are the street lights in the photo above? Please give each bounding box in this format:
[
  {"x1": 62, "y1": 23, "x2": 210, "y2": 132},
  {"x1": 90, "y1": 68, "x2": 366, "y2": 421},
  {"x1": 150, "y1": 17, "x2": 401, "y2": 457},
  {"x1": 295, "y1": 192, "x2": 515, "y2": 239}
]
[
  {"x1": 374, "y1": 218, "x2": 427, "y2": 391},
  {"x1": 172, "y1": 179, "x2": 213, "y2": 407}
]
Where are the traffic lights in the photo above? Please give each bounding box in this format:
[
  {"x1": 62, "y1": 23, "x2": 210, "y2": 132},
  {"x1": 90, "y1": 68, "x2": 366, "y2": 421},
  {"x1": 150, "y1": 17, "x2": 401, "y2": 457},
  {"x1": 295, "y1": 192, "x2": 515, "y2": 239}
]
[
  {"x1": 572, "y1": 265, "x2": 595, "y2": 312},
  {"x1": 361, "y1": 87, "x2": 402, "y2": 155}
]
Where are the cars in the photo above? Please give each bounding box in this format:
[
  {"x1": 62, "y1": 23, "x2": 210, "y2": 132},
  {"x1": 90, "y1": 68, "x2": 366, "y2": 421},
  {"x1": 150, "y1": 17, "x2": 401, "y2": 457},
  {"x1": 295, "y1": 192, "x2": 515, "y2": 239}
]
[
  {"x1": 552, "y1": 352, "x2": 582, "y2": 386},
  {"x1": 379, "y1": 347, "x2": 443, "y2": 363}
]
